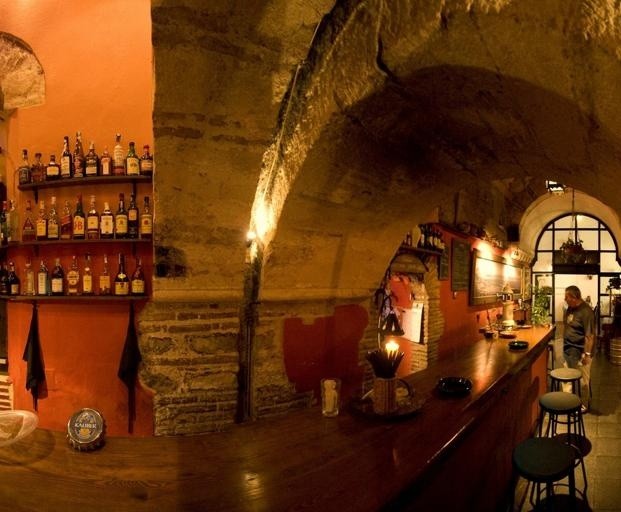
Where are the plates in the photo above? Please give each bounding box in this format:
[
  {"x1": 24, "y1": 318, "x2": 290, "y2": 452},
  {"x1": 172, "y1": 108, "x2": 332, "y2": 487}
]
[
  {"x1": 517, "y1": 325, "x2": 532, "y2": 328},
  {"x1": 438, "y1": 377, "x2": 472, "y2": 396},
  {"x1": 499, "y1": 331, "x2": 518, "y2": 337},
  {"x1": 507, "y1": 340, "x2": 529, "y2": 350}
]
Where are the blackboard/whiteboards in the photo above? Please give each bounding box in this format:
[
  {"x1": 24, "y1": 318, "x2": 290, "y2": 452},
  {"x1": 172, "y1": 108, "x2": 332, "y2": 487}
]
[
  {"x1": 451, "y1": 238, "x2": 471, "y2": 291},
  {"x1": 438, "y1": 245, "x2": 450, "y2": 281}
]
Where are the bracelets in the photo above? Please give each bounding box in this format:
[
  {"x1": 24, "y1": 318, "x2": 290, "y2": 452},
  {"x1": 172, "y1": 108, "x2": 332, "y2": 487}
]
[{"x1": 585, "y1": 352, "x2": 592, "y2": 357}]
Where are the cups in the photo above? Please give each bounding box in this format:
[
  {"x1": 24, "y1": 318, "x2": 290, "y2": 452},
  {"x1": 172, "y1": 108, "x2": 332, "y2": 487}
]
[
  {"x1": 374, "y1": 375, "x2": 398, "y2": 416},
  {"x1": 320, "y1": 377, "x2": 343, "y2": 418}
]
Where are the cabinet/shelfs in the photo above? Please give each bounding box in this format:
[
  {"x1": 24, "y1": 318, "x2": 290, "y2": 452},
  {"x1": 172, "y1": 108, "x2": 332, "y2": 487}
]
[{"x1": 10, "y1": 171, "x2": 153, "y2": 303}]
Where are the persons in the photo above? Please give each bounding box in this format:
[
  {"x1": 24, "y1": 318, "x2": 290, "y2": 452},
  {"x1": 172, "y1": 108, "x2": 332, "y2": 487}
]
[{"x1": 562, "y1": 285, "x2": 596, "y2": 416}]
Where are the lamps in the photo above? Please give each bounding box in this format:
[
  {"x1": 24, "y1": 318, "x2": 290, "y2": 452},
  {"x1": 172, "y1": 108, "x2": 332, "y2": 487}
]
[
  {"x1": 543, "y1": 178, "x2": 569, "y2": 196},
  {"x1": 362, "y1": 293, "x2": 406, "y2": 401}
]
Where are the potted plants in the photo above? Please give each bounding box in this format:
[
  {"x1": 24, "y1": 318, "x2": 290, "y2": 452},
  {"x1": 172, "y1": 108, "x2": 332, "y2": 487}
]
[{"x1": 560, "y1": 237, "x2": 586, "y2": 265}]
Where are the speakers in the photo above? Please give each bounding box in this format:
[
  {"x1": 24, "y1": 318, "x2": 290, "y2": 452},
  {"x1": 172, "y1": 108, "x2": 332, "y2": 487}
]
[{"x1": 505, "y1": 223, "x2": 520, "y2": 242}]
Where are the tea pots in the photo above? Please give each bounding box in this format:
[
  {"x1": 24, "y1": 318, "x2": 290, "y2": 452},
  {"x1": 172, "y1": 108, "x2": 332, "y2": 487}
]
[{"x1": 484, "y1": 331, "x2": 493, "y2": 338}]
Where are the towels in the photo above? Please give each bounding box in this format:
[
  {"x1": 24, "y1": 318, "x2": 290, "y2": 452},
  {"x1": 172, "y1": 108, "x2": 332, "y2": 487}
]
[
  {"x1": 22, "y1": 306, "x2": 47, "y2": 400},
  {"x1": 118, "y1": 306, "x2": 141, "y2": 392}
]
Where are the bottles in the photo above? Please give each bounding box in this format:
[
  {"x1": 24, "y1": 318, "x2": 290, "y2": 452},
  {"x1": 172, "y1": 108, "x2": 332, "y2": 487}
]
[
  {"x1": 0, "y1": 192, "x2": 154, "y2": 297},
  {"x1": 402, "y1": 224, "x2": 444, "y2": 252},
  {"x1": 18, "y1": 131, "x2": 154, "y2": 183}
]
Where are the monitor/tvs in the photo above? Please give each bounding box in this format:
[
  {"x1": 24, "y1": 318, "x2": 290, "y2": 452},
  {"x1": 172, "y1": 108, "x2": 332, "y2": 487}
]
[{"x1": 551, "y1": 250, "x2": 600, "y2": 275}]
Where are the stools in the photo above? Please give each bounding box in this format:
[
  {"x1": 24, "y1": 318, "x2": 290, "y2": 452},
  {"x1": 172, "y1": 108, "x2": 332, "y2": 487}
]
[
  {"x1": 548, "y1": 368, "x2": 584, "y2": 424},
  {"x1": 535, "y1": 391, "x2": 586, "y2": 440},
  {"x1": 511, "y1": 437, "x2": 578, "y2": 511}
]
